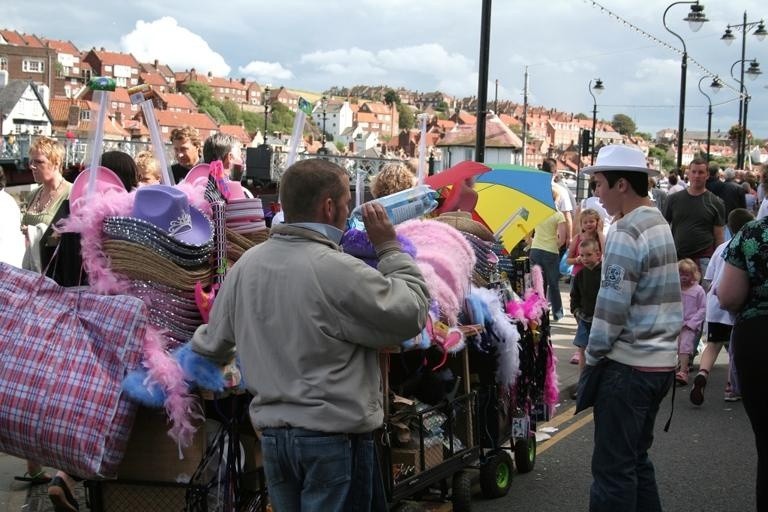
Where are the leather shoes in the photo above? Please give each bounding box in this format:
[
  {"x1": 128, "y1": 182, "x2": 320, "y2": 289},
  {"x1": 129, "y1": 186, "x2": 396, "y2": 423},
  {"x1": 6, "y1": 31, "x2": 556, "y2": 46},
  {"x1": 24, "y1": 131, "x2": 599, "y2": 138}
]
[{"x1": 48, "y1": 476, "x2": 79, "y2": 512}]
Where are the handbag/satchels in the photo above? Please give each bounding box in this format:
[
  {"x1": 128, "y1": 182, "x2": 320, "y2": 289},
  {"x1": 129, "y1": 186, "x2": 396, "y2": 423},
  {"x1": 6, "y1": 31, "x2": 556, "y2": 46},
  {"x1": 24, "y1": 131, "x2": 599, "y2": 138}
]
[{"x1": 0, "y1": 242, "x2": 149, "y2": 481}]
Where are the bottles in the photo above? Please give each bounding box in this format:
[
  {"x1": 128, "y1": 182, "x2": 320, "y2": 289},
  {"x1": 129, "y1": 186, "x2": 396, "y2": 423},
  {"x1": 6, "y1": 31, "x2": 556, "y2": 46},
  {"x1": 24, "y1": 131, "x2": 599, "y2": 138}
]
[{"x1": 344, "y1": 183, "x2": 441, "y2": 234}]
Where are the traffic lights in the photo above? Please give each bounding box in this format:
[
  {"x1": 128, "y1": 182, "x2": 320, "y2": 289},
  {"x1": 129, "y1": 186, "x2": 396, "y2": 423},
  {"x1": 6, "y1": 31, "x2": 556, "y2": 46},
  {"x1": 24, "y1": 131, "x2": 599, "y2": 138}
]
[{"x1": 581, "y1": 129, "x2": 593, "y2": 156}]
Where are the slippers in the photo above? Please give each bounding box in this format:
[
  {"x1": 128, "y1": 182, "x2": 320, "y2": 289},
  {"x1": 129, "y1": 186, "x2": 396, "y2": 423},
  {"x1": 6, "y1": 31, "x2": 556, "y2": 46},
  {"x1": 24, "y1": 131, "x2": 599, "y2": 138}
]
[{"x1": 15, "y1": 471, "x2": 52, "y2": 482}]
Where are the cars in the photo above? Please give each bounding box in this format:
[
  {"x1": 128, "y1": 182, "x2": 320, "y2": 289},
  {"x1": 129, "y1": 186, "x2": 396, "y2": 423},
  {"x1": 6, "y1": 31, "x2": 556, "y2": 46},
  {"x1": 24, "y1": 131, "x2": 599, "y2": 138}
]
[{"x1": 557, "y1": 170, "x2": 576, "y2": 187}]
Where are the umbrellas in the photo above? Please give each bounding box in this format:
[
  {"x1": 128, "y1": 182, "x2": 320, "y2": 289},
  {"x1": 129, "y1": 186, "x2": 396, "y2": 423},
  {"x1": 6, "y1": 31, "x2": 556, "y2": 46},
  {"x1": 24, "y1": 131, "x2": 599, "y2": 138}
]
[{"x1": 416, "y1": 160, "x2": 558, "y2": 254}]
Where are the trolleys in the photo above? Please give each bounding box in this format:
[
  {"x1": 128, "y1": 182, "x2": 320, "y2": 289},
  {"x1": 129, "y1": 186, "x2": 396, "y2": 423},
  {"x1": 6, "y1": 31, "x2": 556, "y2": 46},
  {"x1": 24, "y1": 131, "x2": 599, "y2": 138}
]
[
  {"x1": 83, "y1": 391, "x2": 481, "y2": 512},
  {"x1": 469, "y1": 347, "x2": 536, "y2": 498}
]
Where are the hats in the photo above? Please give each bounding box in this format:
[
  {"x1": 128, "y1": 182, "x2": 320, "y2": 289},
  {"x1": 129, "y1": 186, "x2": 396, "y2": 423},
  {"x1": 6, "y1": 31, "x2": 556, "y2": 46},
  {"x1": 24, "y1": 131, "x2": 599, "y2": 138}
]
[
  {"x1": 582, "y1": 145, "x2": 660, "y2": 175},
  {"x1": 430, "y1": 211, "x2": 500, "y2": 290},
  {"x1": 69, "y1": 164, "x2": 270, "y2": 348}
]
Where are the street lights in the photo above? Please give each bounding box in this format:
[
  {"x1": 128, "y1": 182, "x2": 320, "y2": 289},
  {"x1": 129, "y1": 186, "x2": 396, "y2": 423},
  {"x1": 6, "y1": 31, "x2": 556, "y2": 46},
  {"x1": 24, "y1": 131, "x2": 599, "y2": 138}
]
[
  {"x1": 697, "y1": 74, "x2": 722, "y2": 158},
  {"x1": 315, "y1": 95, "x2": 331, "y2": 153},
  {"x1": 721, "y1": 9, "x2": 767, "y2": 169},
  {"x1": 662, "y1": 0, "x2": 708, "y2": 170},
  {"x1": 729, "y1": 59, "x2": 762, "y2": 170},
  {"x1": 261, "y1": 87, "x2": 270, "y2": 145},
  {"x1": 588, "y1": 77, "x2": 604, "y2": 164}
]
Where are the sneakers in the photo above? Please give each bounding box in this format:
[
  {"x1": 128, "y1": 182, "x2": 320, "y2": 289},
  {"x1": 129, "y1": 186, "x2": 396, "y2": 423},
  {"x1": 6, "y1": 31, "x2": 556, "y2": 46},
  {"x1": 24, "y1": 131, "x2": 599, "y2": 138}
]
[{"x1": 674, "y1": 361, "x2": 742, "y2": 405}]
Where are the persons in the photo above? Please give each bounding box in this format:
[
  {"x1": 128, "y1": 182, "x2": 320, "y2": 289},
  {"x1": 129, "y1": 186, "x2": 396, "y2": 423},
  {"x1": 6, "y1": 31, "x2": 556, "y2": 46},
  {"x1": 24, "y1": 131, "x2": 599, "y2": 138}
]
[
  {"x1": 523, "y1": 159, "x2": 612, "y2": 401},
  {"x1": 648, "y1": 159, "x2": 768, "y2": 511},
  {"x1": 0, "y1": 126, "x2": 246, "y2": 512},
  {"x1": 582, "y1": 144, "x2": 684, "y2": 512},
  {"x1": 192, "y1": 159, "x2": 429, "y2": 512},
  {"x1": 367, "y1": 163, "x2": 417, "y2": 199}
]
